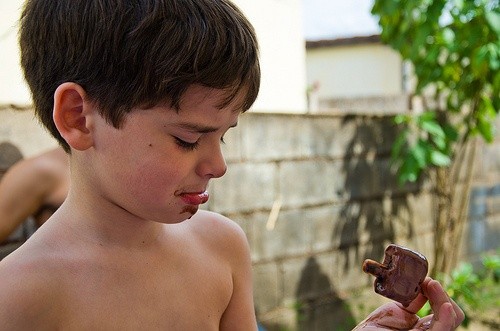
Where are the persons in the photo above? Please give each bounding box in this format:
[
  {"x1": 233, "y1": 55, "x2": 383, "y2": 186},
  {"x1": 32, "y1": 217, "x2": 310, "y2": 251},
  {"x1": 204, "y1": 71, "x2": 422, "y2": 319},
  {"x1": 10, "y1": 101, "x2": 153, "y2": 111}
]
[
  {"x1": 2, "y1": 147, "x2": 75, "y2": 244},
  {"x1": 2, "y1": 0, "x2": 467, "y2": 331}
]
